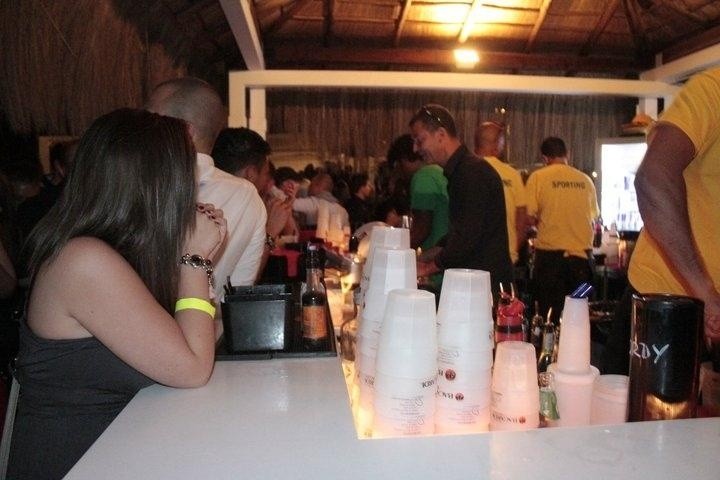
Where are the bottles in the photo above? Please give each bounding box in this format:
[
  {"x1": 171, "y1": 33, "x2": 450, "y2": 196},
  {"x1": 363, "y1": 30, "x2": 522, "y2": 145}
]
[
  {"x1": 628, "y1": 293, "x2": 705, "y2": 421},
  {"x1": 605, "y1": 226, "x2": 621, "y2": 270},
  {"x1": 302, "y1": 245, "x2": 332, "y2": 352},
  {"x1": 529, "y1": 309, "x2": 561, "y2": 427}
]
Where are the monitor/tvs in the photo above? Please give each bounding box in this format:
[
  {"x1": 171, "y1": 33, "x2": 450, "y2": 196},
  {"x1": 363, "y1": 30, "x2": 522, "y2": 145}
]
[{"x1": 594, "y1": 135, "x2": 660, "y2": 238}]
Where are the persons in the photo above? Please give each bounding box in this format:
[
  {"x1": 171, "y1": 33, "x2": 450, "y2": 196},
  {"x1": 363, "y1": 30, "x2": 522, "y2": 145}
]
[
  {"x1": 1, "y1": 75, "x2": 602, "y2": 363},
  {"x1": 598, "y1": 66, "x2": 720, "y2": 376},
  {"x1": 0, "y1": 100, "x2": 230, "y2": 480}
]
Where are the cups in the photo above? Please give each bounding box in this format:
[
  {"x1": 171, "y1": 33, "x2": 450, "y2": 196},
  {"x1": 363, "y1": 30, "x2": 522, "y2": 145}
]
[
  {"x1": 590, "y1": 374, "x2": 628, "y2": 424},
  {"x1": 487, "y1": 340, "x2": 542, "y2": 430},
  {"x1": 355, "y1": 225, "x2": 412, "y2": 330},
  {"x1": 433, "y1": 268, "x2": 495, "y2": 434},
  {"x1": 544, "y1": 296, "x2": 600, "y2": 426},
  {"x1": 356, "y1": 246, "x2": 418, "y2": 433},
  {"x1": 370, "y1": 287, "x2": 436, "y2": 437}
]
